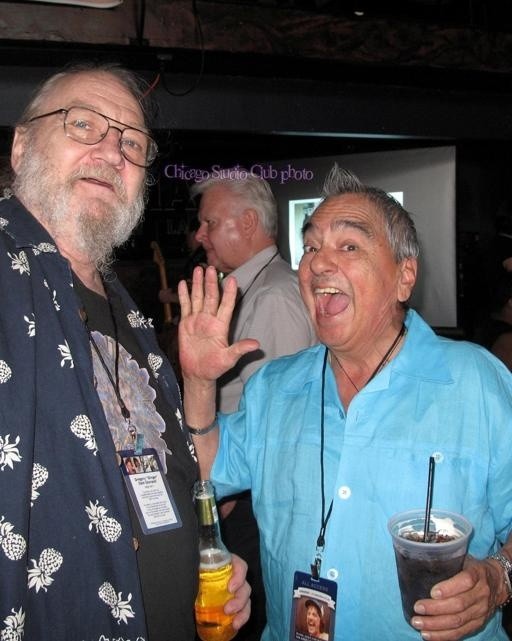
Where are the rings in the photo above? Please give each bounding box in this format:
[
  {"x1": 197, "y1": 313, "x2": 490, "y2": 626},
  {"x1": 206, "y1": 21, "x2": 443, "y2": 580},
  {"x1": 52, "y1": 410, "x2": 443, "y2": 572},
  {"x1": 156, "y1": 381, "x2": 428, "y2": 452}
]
[{"x1": 200, "y1": 308, "x2": 218, "y2": 317}]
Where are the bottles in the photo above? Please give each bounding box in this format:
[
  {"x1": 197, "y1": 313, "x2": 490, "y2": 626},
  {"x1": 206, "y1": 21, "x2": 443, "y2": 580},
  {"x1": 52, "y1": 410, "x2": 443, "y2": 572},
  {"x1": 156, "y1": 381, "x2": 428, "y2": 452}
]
[{"x1": 191, "y1": 478, "x2": 239, "y2": 641}]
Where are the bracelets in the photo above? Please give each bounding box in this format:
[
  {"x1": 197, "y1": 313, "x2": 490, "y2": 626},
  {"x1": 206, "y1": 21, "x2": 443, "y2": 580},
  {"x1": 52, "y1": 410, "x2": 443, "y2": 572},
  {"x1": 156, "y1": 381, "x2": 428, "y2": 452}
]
[{"x1": 182, "y1": 416, "x2": 220, "y2": 439}]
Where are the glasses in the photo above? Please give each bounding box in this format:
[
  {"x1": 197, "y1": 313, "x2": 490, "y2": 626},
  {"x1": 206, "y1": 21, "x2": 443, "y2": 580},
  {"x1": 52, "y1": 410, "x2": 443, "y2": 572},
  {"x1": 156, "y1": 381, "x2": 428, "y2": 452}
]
[{"x1": 30, "y1": 105, "x2": 160, "y2": 168}]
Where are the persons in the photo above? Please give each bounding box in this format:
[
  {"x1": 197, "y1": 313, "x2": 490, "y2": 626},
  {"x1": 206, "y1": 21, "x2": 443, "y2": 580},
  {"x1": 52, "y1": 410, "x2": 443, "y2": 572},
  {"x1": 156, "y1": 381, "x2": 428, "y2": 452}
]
[
  {"x1": 189, "y1": 168, "x2": 321, "y2": 641},
  {"x1": 176, "y1": 160, "x2": 511, "y2": 641},
  {"x1": 157, "y1": 216, "x2": 206, "y2": 387},
  {"x1": 124, "y1": 457, "x2": 134, "y2": 475},
  {"x1": 146, "y1": 457, "x2": 157, "y2": 472},
  {"x1": 0, "y1": 61, "x2": 254, "y2": 641},
  {"x1": 480, "y1": 223, "x2": 510, "y2": 372},
  {"x1": 302, "y1": 598, "x2": 329, "y2": 641},
  {"x1": 131, "y1": 457, "x2": 142, "y2": 473}
]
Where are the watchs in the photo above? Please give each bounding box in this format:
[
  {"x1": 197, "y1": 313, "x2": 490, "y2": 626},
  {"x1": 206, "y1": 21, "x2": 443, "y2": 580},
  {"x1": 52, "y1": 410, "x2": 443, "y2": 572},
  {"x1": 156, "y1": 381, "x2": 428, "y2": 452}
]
[{"x1": 479, "y1": 552, "x2": 511, "y2": 610}]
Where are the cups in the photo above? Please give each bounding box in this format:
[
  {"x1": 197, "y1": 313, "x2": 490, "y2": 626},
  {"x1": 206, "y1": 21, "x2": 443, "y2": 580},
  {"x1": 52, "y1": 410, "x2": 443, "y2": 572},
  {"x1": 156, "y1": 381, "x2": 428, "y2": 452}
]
[{"x1": 386, "y1": 509, "x2": 474, "y2": 629}]
